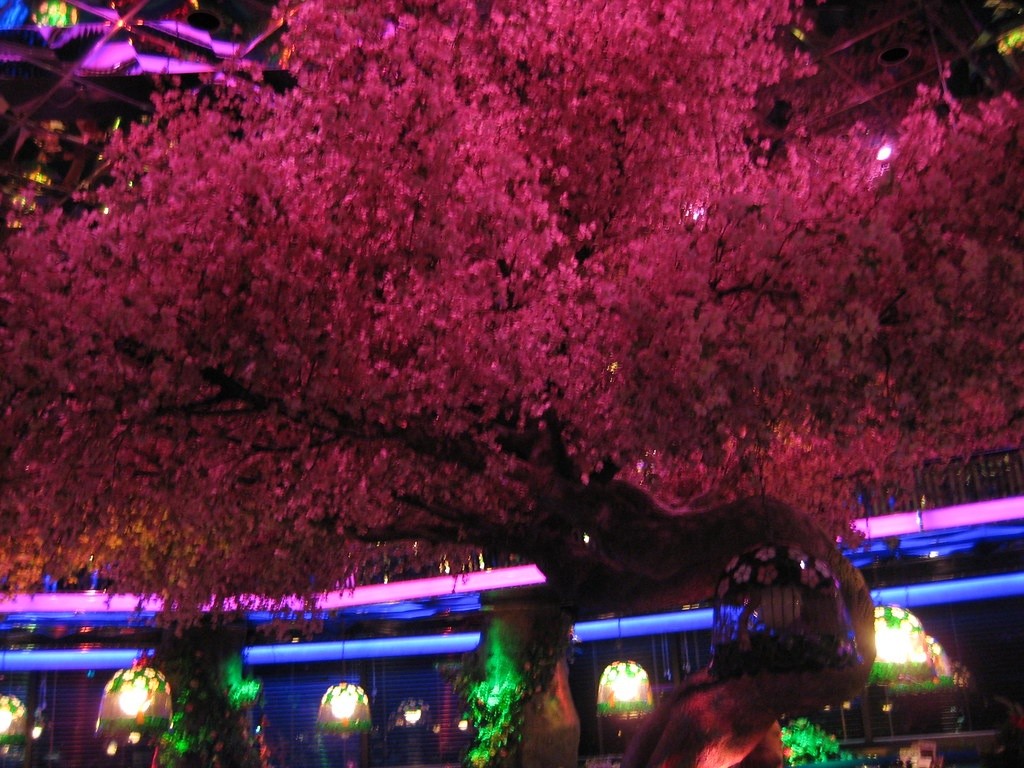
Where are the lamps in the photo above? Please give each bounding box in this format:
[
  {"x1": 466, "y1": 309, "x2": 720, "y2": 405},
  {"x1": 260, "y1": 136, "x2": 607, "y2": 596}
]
[
  {"x1": 872, "y1": 600, "x2": 957, "y2": 696},
  {"x1": 314, "y1": 629, "x2": 372, "y2": 741},
  {"x1": 387, "y1": 689, "x2": 435, "y2": 734},
  {"x1": 704, "y1": 436, "x2": 866, "y2": 678},
  {"x1": 597, "y1": 615, "x2": 652, "y2": 720},
  {"x1": 88, "y1": 657, "x2": 176, "y2": 754},
  {"x1": 0, "y1": 694, "x2": 32, "y2": 762}
]
